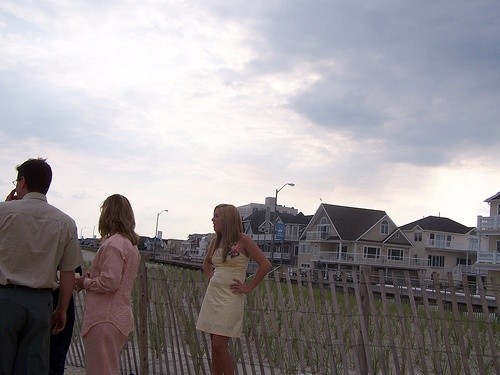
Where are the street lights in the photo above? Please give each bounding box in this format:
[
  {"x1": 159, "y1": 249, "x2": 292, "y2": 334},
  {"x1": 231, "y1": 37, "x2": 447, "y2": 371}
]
[
  {"x1": 269, "y1": 182, "x2": 295, "y2": 280},
  {"x1": 151, "y1": 209, "x2": 169, "y2": 262},
  {"x1": 80, "y1": 227, "x2": 87, "y2": 248},
  {"x1": 465, "y1": 226, "x2": 478, "y2": 293},
  {"x1": 91, "y1": 224, "x2": 99, "y2": 250}
]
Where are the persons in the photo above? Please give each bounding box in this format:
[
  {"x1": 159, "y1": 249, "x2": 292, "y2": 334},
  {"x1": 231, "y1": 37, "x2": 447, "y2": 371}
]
[
  {"x1": 196, "y1": 204, "x2": 271, "y2": 375},
  {"x1": 51, "y1": 259, "x2": 91, "y2": 375},
  {"x1": 75, "y1": 193, "x2": 141, "y2": 375},
  {"x1": 1, "y1": 157, "x2": 79, "y2": 375}
]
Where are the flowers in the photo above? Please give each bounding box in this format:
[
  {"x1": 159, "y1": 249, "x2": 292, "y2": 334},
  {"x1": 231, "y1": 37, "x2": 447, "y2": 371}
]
[{"x1": 231, "y1": 245, "x2": 240, "y2": 255}]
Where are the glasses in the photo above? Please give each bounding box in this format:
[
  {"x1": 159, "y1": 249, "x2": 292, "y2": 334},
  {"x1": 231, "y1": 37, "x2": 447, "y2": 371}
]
[
  {"x1": 100, "y1": 206, "x2": 104, "y2": 212},
  {"x1": 12, "y1": 178, "x2": 22, "y2": 186}
]
